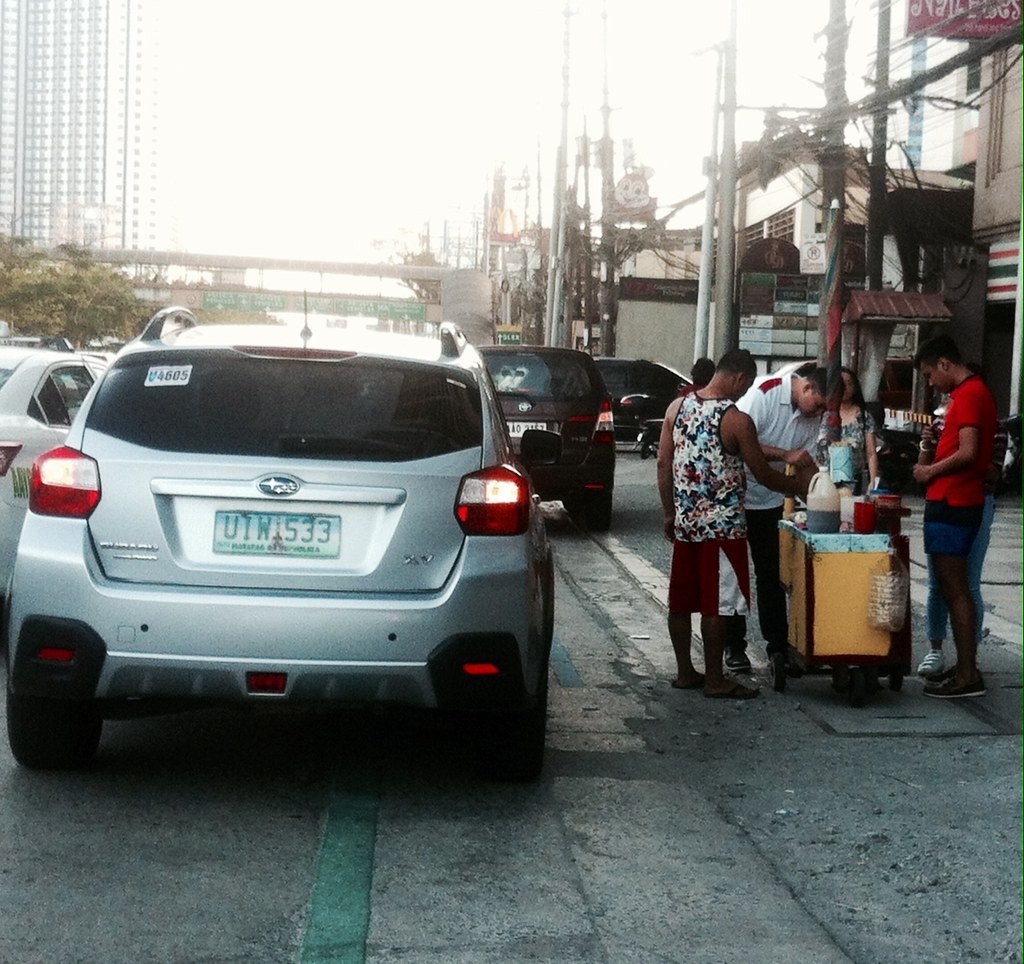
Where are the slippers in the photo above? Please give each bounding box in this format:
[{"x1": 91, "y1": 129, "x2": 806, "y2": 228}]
[
  {"x1": 671, "y1": 672, "x2": 707, "y2": 690},
  {"x1": 705, "y1": 680, "x2": 758, "y2": 700}
]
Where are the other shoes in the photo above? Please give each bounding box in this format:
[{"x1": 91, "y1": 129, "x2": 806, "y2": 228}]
[
  {"x1": 766, "y1": 643, "x2": 786, "y2": 662},
  {"x1": 724, "y1": 641, "x2": 752, "y2": 674}
]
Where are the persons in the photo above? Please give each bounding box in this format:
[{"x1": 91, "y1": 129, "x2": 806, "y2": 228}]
[
  {"x1": 913, "y1": 336, "x2": 1007, "y2": 700},
  {"x1": 656, "y1": 349, "x2": 882, "y2": 699}
]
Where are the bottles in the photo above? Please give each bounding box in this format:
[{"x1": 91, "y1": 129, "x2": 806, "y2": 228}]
[
  {"x1": 876, "y1": 494, "x2": 902, "y2": 536},
  {"x1": 806, "y1": 466, "x2": 841, "y2": 534},
  {"x1": 869, "y1": 489, "x2": 888, "y2": 504},
  {"x1": 864, "y1": 548, "x2": 909, "y2": 632}
]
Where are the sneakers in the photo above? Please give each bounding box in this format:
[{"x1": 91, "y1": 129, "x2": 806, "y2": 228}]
[
  {"x1": 926, "y1": 665, "x2": 957, "y2": 682},
  {"x1": 922, "y1": 669, "x2": 987, "y2": 698},
  {"x1": 917, "y1": 648, "x2": 946, "y2": 674}
]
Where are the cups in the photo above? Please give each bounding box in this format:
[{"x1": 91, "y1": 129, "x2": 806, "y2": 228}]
[{"x1": 853, "y1": 502, "x2": 876, "y2": 534}]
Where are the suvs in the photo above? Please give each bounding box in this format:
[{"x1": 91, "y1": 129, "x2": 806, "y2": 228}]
[
  {"x1": 470, "y1": 345, "x2": 617, "y2": 531},
  {"x1": 0, "y1": 301, "x2": 570, "y2": 784}
]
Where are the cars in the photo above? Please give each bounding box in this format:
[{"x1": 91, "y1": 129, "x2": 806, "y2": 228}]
[
  {"x1": 0, "y1": 332, "x2": 117, "y2": 650},
  {"x1": 591, "y1": 354, "x2": 695, "y2": 443}
]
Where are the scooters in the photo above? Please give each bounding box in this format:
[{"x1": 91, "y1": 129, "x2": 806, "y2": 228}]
[
  {"x1": 619, "y1": 381, "x2": 689, "y2": 461},
  {"x1": 864, "y1": 398, "x2": 1024, "y2": 500}
]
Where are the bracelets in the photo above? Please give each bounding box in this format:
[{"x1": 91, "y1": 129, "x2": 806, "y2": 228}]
[{"x1": 919, "y1": 439, "x2": 932, "y2": 452}]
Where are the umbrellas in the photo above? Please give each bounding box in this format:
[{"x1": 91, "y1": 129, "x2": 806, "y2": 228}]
[{"x1": 824, "y1": 198, "x2": 846, "y2": 447}]
